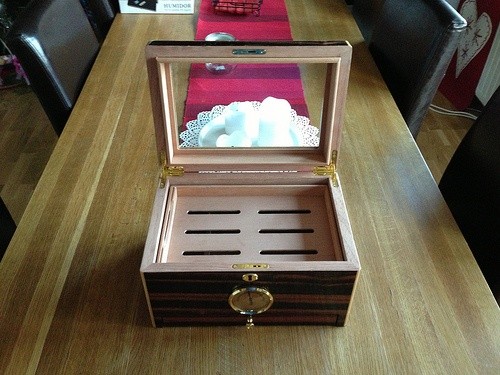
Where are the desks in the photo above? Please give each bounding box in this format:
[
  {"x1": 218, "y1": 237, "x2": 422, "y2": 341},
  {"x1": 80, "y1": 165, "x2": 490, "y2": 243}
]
[{"x1": 0, "y1": 0, "x2": 500, "y2": 374}]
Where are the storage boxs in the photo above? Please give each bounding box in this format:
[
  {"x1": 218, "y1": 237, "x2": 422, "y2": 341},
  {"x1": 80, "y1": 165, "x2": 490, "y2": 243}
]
[{"x1": 138, "y1": 41, "x2": 362, "y2": 329}]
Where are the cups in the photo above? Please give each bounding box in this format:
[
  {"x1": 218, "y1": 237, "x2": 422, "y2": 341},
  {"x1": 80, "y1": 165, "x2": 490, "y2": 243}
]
[{"x1": 204, "y1": 32, "x2": 239, "y2": 74}]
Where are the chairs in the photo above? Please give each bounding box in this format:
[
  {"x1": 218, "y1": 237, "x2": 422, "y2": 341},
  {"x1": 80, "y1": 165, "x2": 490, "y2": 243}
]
[
  {"x1": 4, "y1": 1, "x2": 102, "y2": 140},
  {"x1": 351, "y1": 1, "x2": 471, "y2": 140}
]
